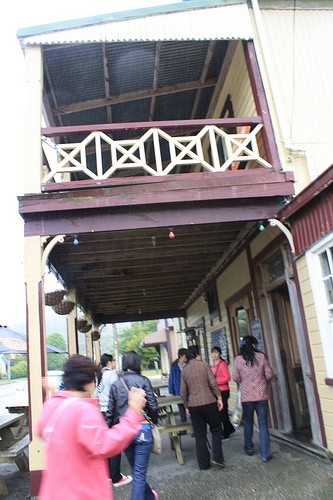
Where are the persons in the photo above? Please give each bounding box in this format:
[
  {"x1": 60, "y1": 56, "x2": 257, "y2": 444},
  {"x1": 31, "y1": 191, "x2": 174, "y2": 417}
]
[
  {"x1": 179, "y1": 346, "x2": 226, "y2": 471},
  {"x1": 95, "y1": 352, "x2": 134, "y2": 487},
  {"x1": 208, "y1": 346, "x2": 238, "y2": 441},
  {"x1": 232, "y1": 335, "x2": 274, "y2": 462},
  {"x1": 107, "y1": 350, "x2": 160, "y2": 500},
  {"x1": 168, "y1": 348, "x2": 196, "y2": 438},
  {"x1": 39, "y1": 354, "x2": 147, "y2": 500}
]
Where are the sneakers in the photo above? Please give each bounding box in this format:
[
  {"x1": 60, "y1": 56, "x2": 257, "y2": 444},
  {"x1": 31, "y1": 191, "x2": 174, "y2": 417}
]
[{"x1": 112, "y1": 473, "x2": 132, "y2": 487}]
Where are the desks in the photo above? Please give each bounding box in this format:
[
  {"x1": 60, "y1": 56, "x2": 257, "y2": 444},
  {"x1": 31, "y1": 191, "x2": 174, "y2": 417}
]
[
  {"x1": 0, "y1": 412, "x2": 30, "y2": 496},
  {"x1": 149, "y1": 379, "x2": 168, "y2": 397},
  {"x1": 5, "y1": 399, "x2": 29, "y2": 439},
  {"x1": 155, "y1": 395, "x2": 187, "y2": 465}
]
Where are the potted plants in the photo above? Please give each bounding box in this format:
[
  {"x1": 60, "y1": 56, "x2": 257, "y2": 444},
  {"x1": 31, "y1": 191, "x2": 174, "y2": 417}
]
[
  {"x1": 44, "y1": 289, "x2": 67, "y2": 306},
  {"x1": 79, "y1": 323, "x2": 92, "y2": 334},
  {"x1": 51, "y1": 298, "x2": 75, "y2": 315},
  {"x1": 76, "y1": 318, "x2": 87, "y2": 330},
  {"x1": 92, "y1": 331, "x2": 100, "y2": 341}
]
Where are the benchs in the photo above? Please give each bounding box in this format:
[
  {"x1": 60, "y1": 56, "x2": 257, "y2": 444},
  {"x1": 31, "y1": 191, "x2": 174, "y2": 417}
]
[
  {"x1": 158, "y1": 423, "x2": 192, "y2": 434},
  {"x1": 0, "y1": 432, "x2": 31, "y2": 458}
]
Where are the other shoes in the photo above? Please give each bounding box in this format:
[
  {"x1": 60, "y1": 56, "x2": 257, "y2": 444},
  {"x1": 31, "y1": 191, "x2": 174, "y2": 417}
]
[
  {"x1": 211, "y1": 461, "x2": 224, "y2": 467},
  {"x1": 177, "y1": 429, "x2": 186, "y2": 435},
  {"x1": 245, "y1": 448, "x2": 253, "y2": 456},
  {"x1": 227, "y1": 428, "x2": 235, "y2": 434},
  {"x1": 220, "y1": 434, "x2": 229, "y2": 438},
  {"x1": 152, "y1": 489, "x2": 158, "y2": 500}
]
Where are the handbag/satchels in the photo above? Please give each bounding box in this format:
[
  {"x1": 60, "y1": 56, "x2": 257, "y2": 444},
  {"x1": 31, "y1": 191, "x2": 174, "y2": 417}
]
[{"x1": 151, "y1": 425, "x2": 161, "y2": 454}]
